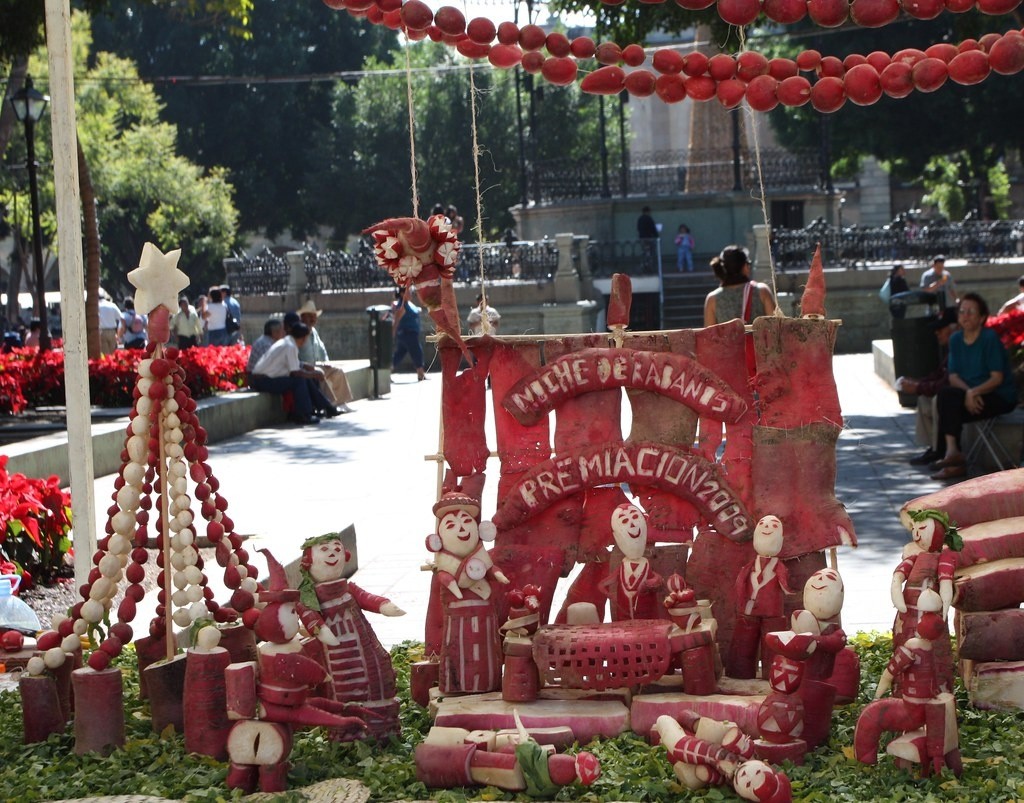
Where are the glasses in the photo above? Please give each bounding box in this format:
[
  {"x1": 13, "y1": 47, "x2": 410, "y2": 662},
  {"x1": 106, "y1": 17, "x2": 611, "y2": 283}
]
[{"x1": 958, "y1": 306, "x2": 979, "y2": 315}]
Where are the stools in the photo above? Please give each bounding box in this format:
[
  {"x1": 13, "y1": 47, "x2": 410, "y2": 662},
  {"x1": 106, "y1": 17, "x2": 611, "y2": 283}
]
[{"x1": 964, "y1": 411, "x2": 1016, "y2": 472}]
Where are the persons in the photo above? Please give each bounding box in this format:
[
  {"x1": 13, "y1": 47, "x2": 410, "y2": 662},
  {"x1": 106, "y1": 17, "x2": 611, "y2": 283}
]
[
  {"x1": 0, "y1": 283, "x2": 355, "y2": 425},
  {"x1": 251, "y1": 491, "x2": 967, "y2": 802},
  {"x1": 880, "y1": 212, "x2": 1024, "y2": 479},
  {"x1": 387, "y1": 284, "x2": 430, "y2": 382},
  {"x1": 635, "y1": 207, "x2": 661, "y2": 272},
  {"x1": 673, "y1": 224, "x2": 696, "y2": 273},
  {"x1": 466, "y1": 294, "x2": 500, "y2": 389},
  {"x1": 429, "y1": 203, "x2": 520, "y2": 283},
  {"x1": 702, "y1": 246, "x2": 782, "y2": 423}
]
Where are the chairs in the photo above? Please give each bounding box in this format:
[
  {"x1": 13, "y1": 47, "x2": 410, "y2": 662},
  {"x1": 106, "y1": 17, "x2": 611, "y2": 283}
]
[
  {"x1": 234, "y1": 235, "x2": 559, "y2": 294},
  {"x1": 770, "y1": 221, "x2": 1024, "y2": 268}
]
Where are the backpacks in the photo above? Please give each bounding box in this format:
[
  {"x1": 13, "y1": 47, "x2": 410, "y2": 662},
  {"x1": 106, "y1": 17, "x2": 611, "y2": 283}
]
[{"x1": 126, "y1": 312, "x2": 143, "y2": 333}]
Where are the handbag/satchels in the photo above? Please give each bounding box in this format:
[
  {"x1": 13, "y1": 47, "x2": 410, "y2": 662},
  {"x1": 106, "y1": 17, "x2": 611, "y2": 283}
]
[
  {"x1": 879, "y1": 277, "x2": 891, "y2": 303},
  {"x1": 227, "y1": 312, "x2": 241, "y2": 333}
]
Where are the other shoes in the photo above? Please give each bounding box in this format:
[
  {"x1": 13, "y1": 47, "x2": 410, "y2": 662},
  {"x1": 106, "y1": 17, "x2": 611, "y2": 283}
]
[
  {"x1": 289, "y1": 404, "x2": 359, "y2": 424},
  {"x1": 929, "y1": 462, "x2": 965, "y2": 479},
  {"x1": 909, "y1": 446, "x2": 945, "y2": 466},
  {"x1": 936, "y1": 451, "x2": 963, "y2": 465}
]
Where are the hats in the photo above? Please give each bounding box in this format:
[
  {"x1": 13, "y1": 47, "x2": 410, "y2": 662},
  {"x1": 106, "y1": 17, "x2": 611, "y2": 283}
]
[{"x1": 298, "y1": 300, "x2": 321, "y2": 320}]
